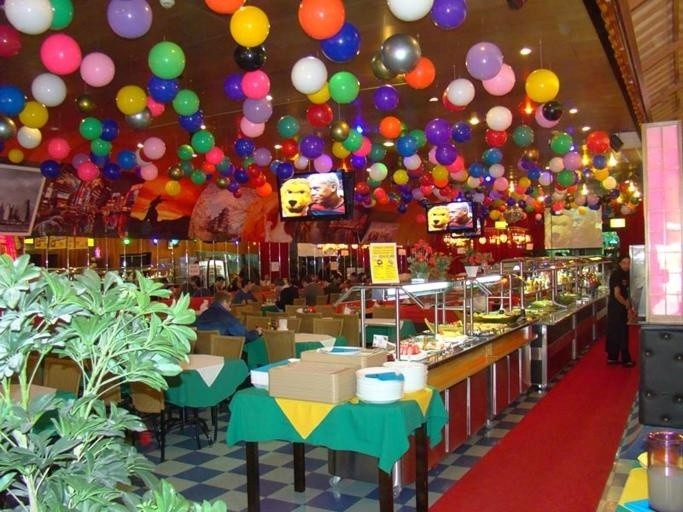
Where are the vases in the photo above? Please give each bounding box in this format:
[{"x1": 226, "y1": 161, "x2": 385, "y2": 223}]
[
  {"x1": 437, "y1": 271, "x2": 448, "y2": 281},
  {"x1": 409, "y1": 270, "x2": 430, "y2": 284},
  {"x1": 464, "y1": 266, "x2": 480, "y2": 279}
]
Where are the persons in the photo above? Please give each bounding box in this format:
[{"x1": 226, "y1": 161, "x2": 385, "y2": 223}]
[
  {"x1": 447, "y1": 202, "x2": 472, "y2": 228},
  {"x1": 195, "y1": 292, "x2": 263, "y2": 343},
  {"x1": 606, "y1": 255, "x2": 634, "y2": 366},
  {"x1": 306, "y1": 172, "x2": 345, "y2": 216},
  {"x1": 209, "y1": 272, "x2": 372, "y2": 311},
  {"x1": 447, "y1": 253, "x2": 469, "y2": 281},
  {"x1": 568, "y1": 207, "x2": 601, "y2": 248}
]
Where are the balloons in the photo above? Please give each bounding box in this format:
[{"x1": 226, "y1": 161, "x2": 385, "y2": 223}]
[
  {"x1": 445, "y1": 42, "x2": 643, "y2": 216},
  {"x1": 1, "y1": 0, "x2": 465, "y2": 208}
]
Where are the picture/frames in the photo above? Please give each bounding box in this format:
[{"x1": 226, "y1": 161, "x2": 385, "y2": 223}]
[{"x1": 0, "y1": 163, "x2": 46, "y2": 237}]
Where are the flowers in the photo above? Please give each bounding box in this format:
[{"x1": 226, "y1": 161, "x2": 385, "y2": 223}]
[{"x1": 404, "y1": 238, "x2": 493, "y2": 274}]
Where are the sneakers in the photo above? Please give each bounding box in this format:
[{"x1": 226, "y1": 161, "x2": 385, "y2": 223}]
[
  {"x1": 608, "y1": 360, "x2": 619, "y2": 364},
  {"x1": 622, "y1": 361, "x2": 633, "y2": 367}
]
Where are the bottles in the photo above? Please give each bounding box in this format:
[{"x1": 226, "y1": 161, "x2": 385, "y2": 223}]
[{"x1": 647, "y1": 433, "x2": 682, "y2": 512}]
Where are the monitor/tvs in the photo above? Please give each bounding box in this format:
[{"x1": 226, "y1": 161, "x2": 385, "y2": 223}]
[
  {"x1": 425, "y1": 200, "x2": 475, "y2": 233},
  {"x1": 277, "y1": 168, "x2": 348, "y2": 223}
]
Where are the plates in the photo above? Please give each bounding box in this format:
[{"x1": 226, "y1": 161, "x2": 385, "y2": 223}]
[{"x1": 354, "y1": 361, "x2": 427, "y2": 404}]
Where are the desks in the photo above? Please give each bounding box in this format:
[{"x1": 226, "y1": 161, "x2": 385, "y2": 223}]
[
  {"x1": 359, "y1": 317, "x2": 412, "y2": 345},
  {"x1": 235, "y1": 387, "x2": 436, "y2": 512}
]
